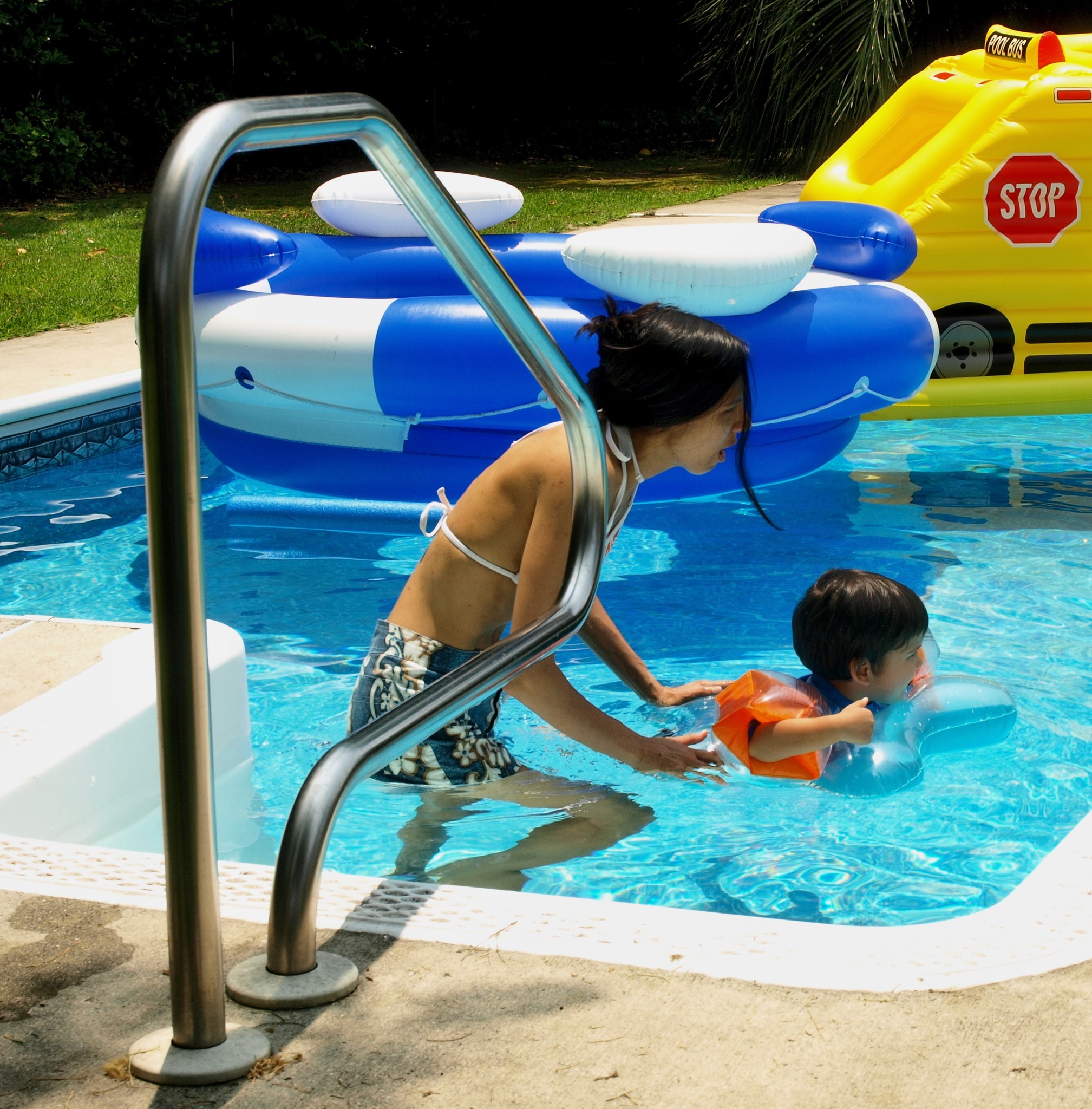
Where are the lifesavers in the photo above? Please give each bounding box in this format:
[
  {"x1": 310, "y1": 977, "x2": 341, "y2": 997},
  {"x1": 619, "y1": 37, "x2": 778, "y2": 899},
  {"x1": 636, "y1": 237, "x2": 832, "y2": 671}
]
[
  {"x1": 193, "y1": 168, "x2": 942, "y2": 501},
  {"x1": 634, "y1": 678, "x2": 1016, "y2": 798}
]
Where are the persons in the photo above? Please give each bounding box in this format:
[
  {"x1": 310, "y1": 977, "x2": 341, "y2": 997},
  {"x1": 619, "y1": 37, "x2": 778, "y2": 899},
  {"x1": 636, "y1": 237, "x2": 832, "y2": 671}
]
[
  {"x1": 749, "y1": 568, "x2": 929, "y2": 763},
  {"x1": 345, "y1": 298, "x2": 784, "y2": 788}
]
